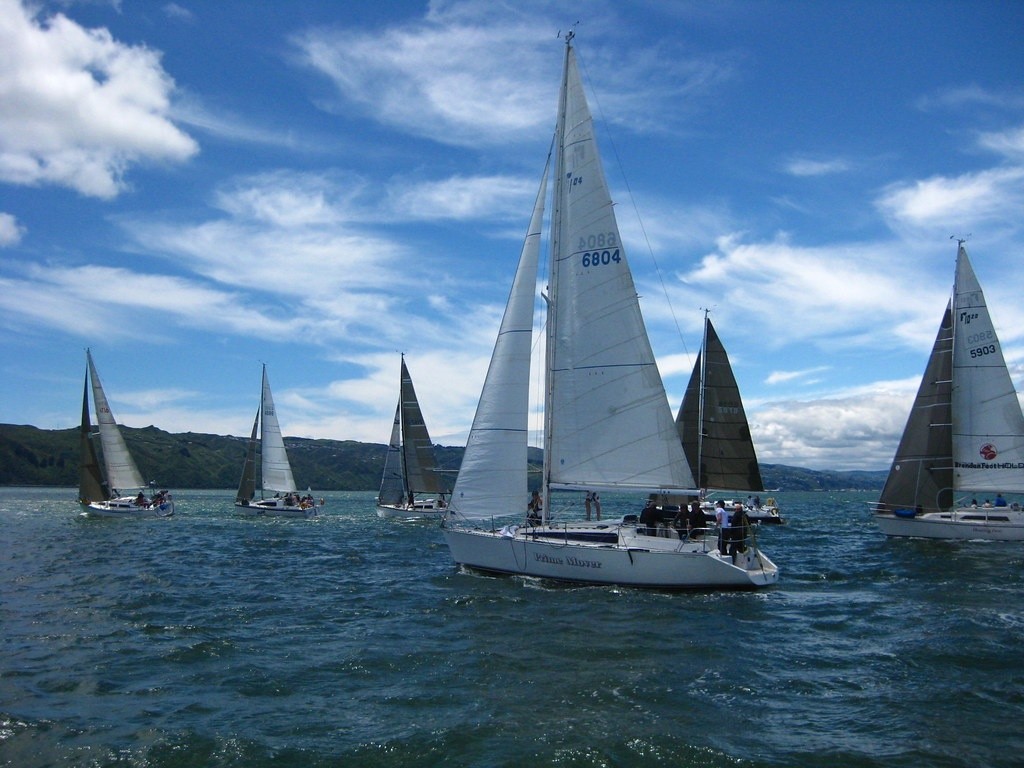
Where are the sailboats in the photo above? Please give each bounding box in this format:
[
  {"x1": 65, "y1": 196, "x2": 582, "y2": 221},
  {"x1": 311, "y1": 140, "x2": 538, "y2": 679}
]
[
  {"x1": 673, "y1": 306, "x2": 787, "y2": 525},
  {"x1": 868, "y1": 238, "x2": 1024, "y2": 541},
  {"x1": 441, "y1": 29, "x2": 780, "y2": 586},
  {"x1": 375, "y1": 351, "x2": 459, "y2": 519},
  {"x1": 234, "y1": 362, "x2": 331, "y2": 520},
  {"x1": 76, "y1": 346, "x2": 176, "y2": 520}
]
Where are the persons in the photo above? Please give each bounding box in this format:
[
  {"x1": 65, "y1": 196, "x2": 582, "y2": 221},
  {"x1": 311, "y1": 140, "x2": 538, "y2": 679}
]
[
  {"x1": 526, "y1": 490, "x2": 543, "y2": 526},
  {"x1": 405, "y1": 490, "x2": 415, "y2": 510},
  {"x1": 971, "y1": 493, "x2": 1024, "y2": 512},
  {"x1": 640, "y1": 501, "x2": 665, "y2": 536},
  {"x1": 134, "y1": 490, "x2": 168, "y2": 508},
  {"x1": 673, "y1": 496, "x2": 760, "y2": 565},
  {"x1": 585, "y1": 490, "x2": 601, "y2": 521},
  {"x1": 273, "y1": 492, "x2": 324, "y2": 511}
]
